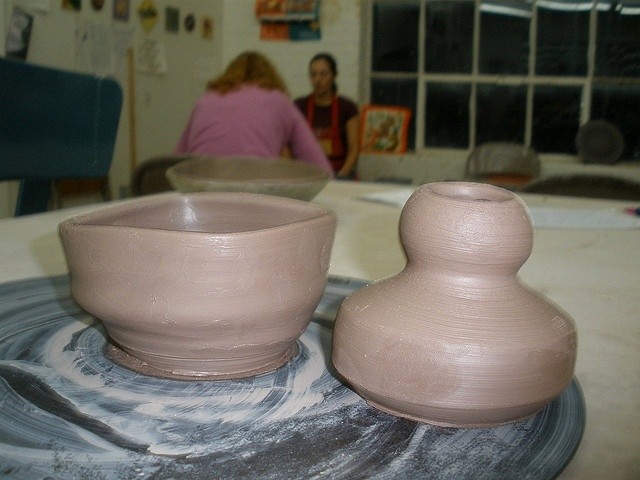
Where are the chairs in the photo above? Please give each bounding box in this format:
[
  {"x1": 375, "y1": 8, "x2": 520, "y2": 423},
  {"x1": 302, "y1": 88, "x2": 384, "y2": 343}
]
[{"x1": 0, "y1": 59, "x2": 122, "y2": 217}]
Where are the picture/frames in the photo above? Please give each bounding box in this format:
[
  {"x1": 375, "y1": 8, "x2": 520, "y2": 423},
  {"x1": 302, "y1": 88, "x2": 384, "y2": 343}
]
[{"x1": 359, "y1": 105, "x2": 411, "y2": 154}]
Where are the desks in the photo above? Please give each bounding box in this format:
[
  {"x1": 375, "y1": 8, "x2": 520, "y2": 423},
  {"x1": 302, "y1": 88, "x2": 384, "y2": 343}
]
[{"x1": 0, "y1": 180, "x2": 640, "y2": 479}]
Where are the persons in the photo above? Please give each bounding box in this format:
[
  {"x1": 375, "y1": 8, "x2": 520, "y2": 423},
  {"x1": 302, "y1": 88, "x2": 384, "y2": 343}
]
[
  {"x1": 176, "y1": 50, "x2": 337, "y2": 180},
  {"x1": 280, "y1": 52, "x2": 360, "y2": 181}
]
